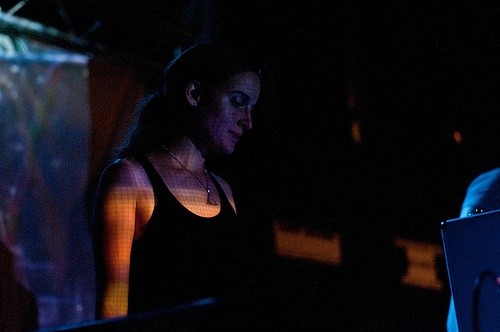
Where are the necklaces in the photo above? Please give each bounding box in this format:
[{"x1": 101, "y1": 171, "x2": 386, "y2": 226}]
[{"x1": 158, "y1": 142, "x2": 217, "y2": 205}]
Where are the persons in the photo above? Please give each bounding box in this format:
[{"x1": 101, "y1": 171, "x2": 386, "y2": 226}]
[
  {"x1": 92, "y1": 41, "x2": 269, "y2": 320},
  {"x1": 445, "y1": 166, "x2": 500, "y2": 331}
]
